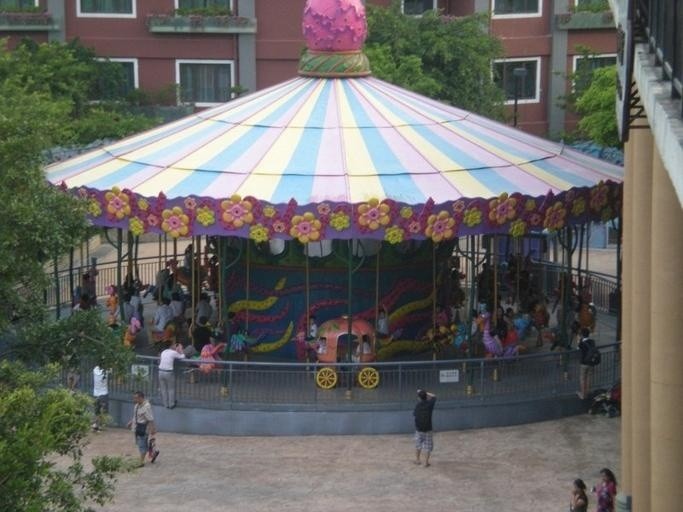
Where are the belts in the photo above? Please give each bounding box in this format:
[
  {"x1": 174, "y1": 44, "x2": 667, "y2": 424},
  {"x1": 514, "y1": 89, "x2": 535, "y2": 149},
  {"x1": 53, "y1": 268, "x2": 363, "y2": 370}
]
[{"x1": 158, "y1": 368, "x2": 174, "y2": 373}]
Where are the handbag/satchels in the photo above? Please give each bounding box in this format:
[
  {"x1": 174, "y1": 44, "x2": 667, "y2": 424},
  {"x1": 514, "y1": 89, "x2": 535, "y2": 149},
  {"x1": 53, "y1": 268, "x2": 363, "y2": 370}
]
[{"x1": 134, "y1": 422, "x2": 147, "y2": 435}]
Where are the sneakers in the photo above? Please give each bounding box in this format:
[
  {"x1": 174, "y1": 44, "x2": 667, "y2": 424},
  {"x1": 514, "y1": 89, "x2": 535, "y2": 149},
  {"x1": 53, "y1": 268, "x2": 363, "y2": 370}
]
[
  {"x1": 134, "y1": 449, "x2": 160, "y2": 469},
  {"x1": 166, "y1": 400, "x2": 178, "y2": 410},
  {"x1": 412, "y1": 460, "x2": 431, "y2": 468}
]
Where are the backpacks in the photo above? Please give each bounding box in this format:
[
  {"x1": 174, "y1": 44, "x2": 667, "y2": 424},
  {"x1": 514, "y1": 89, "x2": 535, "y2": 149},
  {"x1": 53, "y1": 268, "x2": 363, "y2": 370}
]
[{"x1": 581, "y1": 339, "x2": 601, "y2": 365}]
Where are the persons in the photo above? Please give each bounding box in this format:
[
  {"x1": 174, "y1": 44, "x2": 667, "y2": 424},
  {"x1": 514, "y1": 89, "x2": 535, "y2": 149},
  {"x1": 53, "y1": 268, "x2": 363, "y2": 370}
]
[
  {"x1": 125, "y1": 390, "x2": 159, "y2": 470},
  {"x1": 72, "y1": 242, "x2": 247, "y2": 374},
  {"x1": 411, "y1": 388, "x2": 437, "y2": 466},
  {"x1": 592, "y1": 468, "x2": 618, "y2": 511},
  {"x1": 432, "y1": 248, "x2": 596, "y2": 372},
  {"x1": 91, "y1": 357, "x2": 118, "y2": 433},
  {"x1": 574, "y1": 327, "x2": 595, "y2": 398},
  {"x1": 303, "y1": 303, "x2": 391, "y2": 373},
  {"x1": 158, "y1": 339, "x2": 185, "y2": 409},
  {"x1": 568, "y1": 477, "x2": 589, "y2": 512}
]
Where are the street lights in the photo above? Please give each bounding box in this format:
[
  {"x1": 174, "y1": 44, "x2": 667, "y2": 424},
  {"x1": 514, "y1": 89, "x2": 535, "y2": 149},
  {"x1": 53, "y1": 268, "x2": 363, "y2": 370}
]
[{"x1": 513, "y1": 68, "x2": 527, "y2": 128}]
